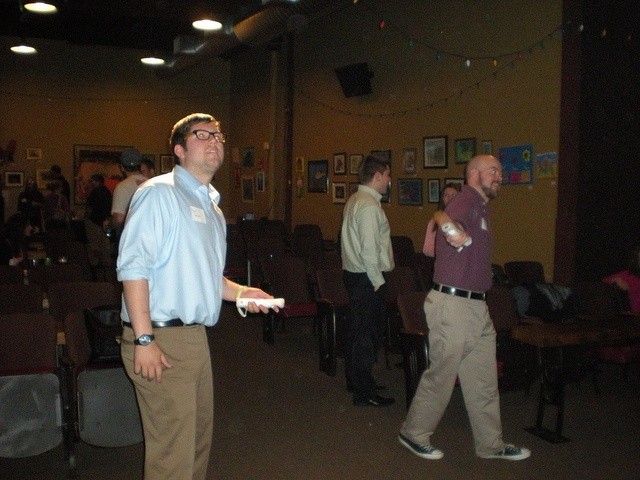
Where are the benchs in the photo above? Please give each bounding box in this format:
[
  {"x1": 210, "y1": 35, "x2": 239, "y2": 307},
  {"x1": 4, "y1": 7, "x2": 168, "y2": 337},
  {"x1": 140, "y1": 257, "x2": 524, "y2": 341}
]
[{"x1": 508, "y1": 315, "x2": 639, "y2": 444}]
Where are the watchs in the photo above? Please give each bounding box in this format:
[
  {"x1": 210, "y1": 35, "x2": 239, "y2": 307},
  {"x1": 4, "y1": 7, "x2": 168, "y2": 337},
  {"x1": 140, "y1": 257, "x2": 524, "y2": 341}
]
[{"x1": 133, "y1": 334, "x2": 155, "y2": 346}]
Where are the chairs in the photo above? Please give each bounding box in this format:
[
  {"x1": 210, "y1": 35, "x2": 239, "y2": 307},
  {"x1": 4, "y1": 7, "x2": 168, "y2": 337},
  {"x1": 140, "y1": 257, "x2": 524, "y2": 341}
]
[
  {"x1": 64, "y1": 310, "x2": 92, "y2": 446},
  {"x1": 485, "y1": 290, "x2": 523, "y2": 391},
  {"x1": 503, "y1": 260, "x2": 544, "y2": 290},
  {"x1": 303, "y1": 254, "x2": 344, "y2": 334},
  {"x1": 1, "y1": 312, "x2": 72, "y2": 476},
  {"x1": 258, "y1": 258, "x2": 319, "y2": 343},
  {"x1": 391, "y1": 234, "x2": 433, "y2": 289},
  {"x1": 398, "y1": 291, "x2": 431, "y2": 410},
  {"x1": 384, "y1": 270, "x2": 420, "y2": 365},
  {"x1": 226, "y1": 221, "x2": 341, "y2": 283},
  {"x1": 315, "y1": 268, "x2": 349, "y2": 378},
  {"x1": 2, "y1": 212, "x2": 121, "y2": 317}
]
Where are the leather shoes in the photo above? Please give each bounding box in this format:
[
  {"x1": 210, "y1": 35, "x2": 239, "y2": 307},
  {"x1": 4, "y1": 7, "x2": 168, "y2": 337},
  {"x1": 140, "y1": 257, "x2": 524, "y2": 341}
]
[{"x1": 349, "y1": 380, "x2": 394, "y2": 407}]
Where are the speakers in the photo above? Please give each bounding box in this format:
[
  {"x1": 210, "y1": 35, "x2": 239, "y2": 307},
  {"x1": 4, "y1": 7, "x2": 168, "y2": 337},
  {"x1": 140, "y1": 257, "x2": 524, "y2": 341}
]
[{"x1": 334, "y1": 61, "x2": 372, "y2": 98}]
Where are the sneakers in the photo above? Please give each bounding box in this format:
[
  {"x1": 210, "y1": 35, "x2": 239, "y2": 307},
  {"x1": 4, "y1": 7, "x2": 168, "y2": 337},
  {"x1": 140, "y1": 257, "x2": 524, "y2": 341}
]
[
  {"x1": 479, "y1": 443, "x2": 531, "y2": 460},
  {"x1": 398, "y1": 432, "x2": 443, "y2": 459}
]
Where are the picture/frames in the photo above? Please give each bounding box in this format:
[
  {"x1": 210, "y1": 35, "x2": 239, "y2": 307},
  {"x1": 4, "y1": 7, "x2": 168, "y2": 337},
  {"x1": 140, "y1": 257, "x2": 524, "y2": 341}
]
[
  {"x1": 482, "y1": 139, "x2": 493, "y2": 153},
  {"x1": 5, "y1": 171, "x2": 23, "y2": 186},
  {"x1": 454, "y1": 138, "x2": 476, "y2": 164},
  {"x1": 397, "y1": 178, "x2": 422, "y2": 205},
  {"x1": 403, "y1": 147, "x2": 416, "y2": 174},
  {"x1": 242, "y1": 146, "x2": 257, "y2": 168},
  {"x1": 380, "y1": 189, "x2": 389, "y2": 202},
  {"x1": 308, "y1": 160, "x2": 328, "y2": 193},
  {"x1": 370, "y1": 150, "x2": 391, "y2": 165},
  {"x1": 349, "y1": 154, "x2": 365, "y2": 175},
  {"x1": 240, "y1": 175, "x2": 255, "y2": 203},
  {"x1": 73, "y1": 143, "x2": 135, "y2": 204},
  {"x1": 427, "y1": 178, "x2": 440, "y2": 204},
  {"x1": 333, "y1": 153, "x2": 346, "y2": 174},
  {"x1": 422, "y1": 135, "x2": 448, "y2": 169},
  {"x1": 26, "y1": 146, "x2": 42, "y2": 160},
  {"x1": 332, "y1": 182, "x2": 347, "y2": 204},
  {"x1": 159, "y1": 153, "x2": 174, "y2": 173},
  {"x1": 444, "y1": 177, "x2": 466, "y2": 189},
  {"x1": 36, "y1": 168, "x2": 52, "y2": 188},
  {"x1": 349, "y1": 182, "x2": 359, "y2": 192}
]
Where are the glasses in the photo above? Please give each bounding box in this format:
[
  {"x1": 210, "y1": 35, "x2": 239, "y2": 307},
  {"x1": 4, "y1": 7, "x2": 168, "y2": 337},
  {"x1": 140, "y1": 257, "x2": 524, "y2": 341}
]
[{"x1": 185, "y1": 130, "x2": 226, "y2": 143}]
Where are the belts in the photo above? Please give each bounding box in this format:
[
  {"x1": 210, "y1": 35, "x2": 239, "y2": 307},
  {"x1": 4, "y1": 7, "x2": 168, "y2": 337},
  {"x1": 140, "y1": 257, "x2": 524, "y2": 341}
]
[
  {"x1": 120, "y1": 318, "x2": 199, "y2": 329},
  {"x1": 430, "y1": 281, "x2": 488, "y2": 300}
]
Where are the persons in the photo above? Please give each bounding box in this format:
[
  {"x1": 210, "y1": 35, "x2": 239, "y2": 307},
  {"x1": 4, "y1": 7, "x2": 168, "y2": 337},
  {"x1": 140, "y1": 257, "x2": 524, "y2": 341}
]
[
  {"x1": 115, "y1": 112, "x2": 281, "y2": 480},
  {"x1": 110, "y1": 148, "x2": 148, "y2": 231},
  {"x1": 2, "y1": 159, "x2": 25, "y2": 213},
  {"x1": 441, "y1": 184, "x2": 459, "y2": 206},
  {"x1": 18, "y1": 176, "x2": 44, "y2": 211},
  {"x1": 82, "y1": 173, "x2": 113, "y2": 282},
  {"x1": 398, "y1": 153, "x2": 532, "y2": 461},
  {"x1": 47, "y1": 164, "x2": 71, "y2": 206},
  {"x1": 140, "y1": 159, "x2": 156, "y2": 180},
  {"x1": 341, "y1": 156, "x2": 396, "y2": 407},
  {"x1": 46, "y1": 180, "x2": 69, "y2": 210},
  {"x1": 1, "y1": 214, "x2": 33, "y2": 267}
]
